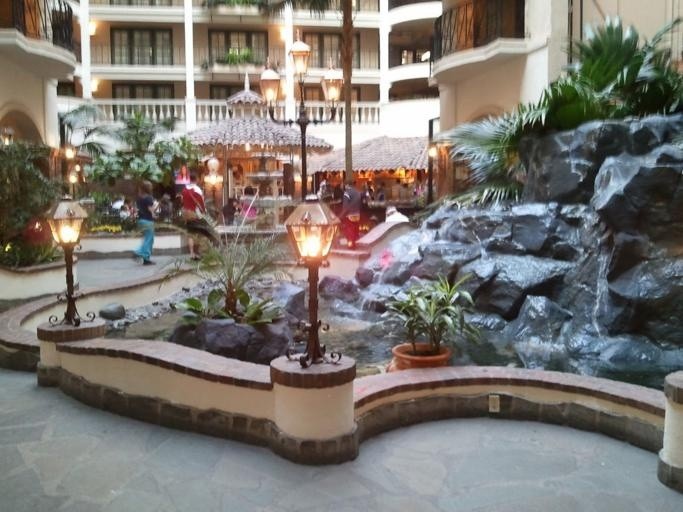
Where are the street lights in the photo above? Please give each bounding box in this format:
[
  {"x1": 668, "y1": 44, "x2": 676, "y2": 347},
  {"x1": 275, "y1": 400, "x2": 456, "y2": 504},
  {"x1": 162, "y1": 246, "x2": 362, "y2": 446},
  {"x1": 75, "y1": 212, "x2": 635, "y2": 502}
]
[
  {"x1": 44, "y1": 194, "x2": 96, "y2": 327},
  {"x1": 204, "y1": 158, "x2": 223, "y2": 205},
  {"x1": 285, "y1": 194, "x2": 344, "y2": 367},
  {"x1": 256, "y1": 27, "x2": 344, "y2": 200}
]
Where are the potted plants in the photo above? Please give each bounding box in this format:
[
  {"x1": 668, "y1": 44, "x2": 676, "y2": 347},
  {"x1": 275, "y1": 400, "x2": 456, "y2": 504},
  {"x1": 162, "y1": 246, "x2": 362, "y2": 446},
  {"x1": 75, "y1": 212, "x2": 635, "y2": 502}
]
[{"x1": 386, "y1": 273, "x2": 476, "y2": 373}]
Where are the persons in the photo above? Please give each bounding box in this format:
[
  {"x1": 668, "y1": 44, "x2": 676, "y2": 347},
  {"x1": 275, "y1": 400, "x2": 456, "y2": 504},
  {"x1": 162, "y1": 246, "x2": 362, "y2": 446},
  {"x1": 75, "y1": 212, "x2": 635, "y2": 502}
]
[
  {"x1": 318, "y1": 178, "x2": 335, "y2": 203},
  {"x1": 133, "y1": 180, "x2": 156, "y2": 266},
  {"x1": 112, "y1": 193, "x2": 183, "y2": 224},
  {"x1": 237, "y1": 185, "x2": 257, "y2": 227},
  {"x1": 179, "y1": 173, "x2": 207, "y2": 261},
  {"x1": 334, "y1": 179, "x2": 422, "y2": 201},
  {"x1": 174, "y1": 164, "x2": 189, "y2": 184},
  {"x1": 338, "y1": 179, "x2": 362, "y2": 248},
  {"x1": 223, "y1": 198, "x2": 237, "y2": 226}
]
